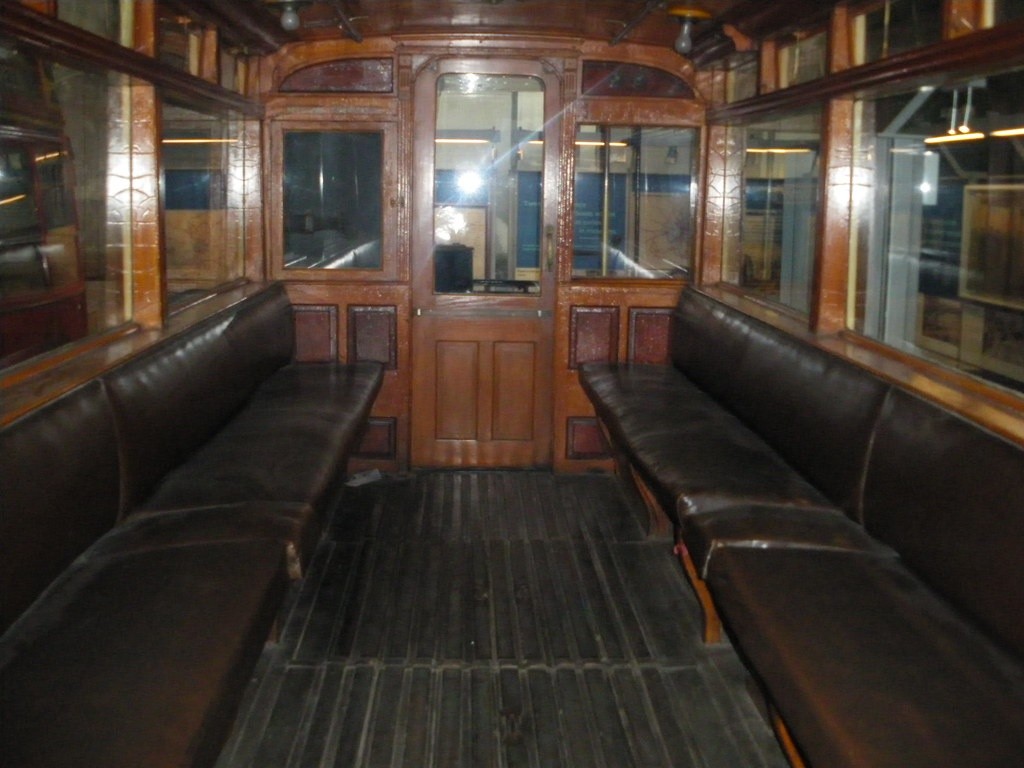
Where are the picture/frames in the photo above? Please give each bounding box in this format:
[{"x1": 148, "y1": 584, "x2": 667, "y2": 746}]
[
  {"x1": 958, "y1": 184, "x2": 1024, "y2": 312},
  {"x1": 917, "y1": 291, "x2": 961, "y2": 359},
  {"x1": 959, "y1": 303, "x2": 1024, "y2": 381}
]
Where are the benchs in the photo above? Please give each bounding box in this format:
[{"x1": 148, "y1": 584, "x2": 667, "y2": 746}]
[
  {"x1": 0, "y1": 281, "x2": 384, "y2": 768},
  {"x1": 580, "y1": 288, "x2": 1024, "y2": 768}
]
